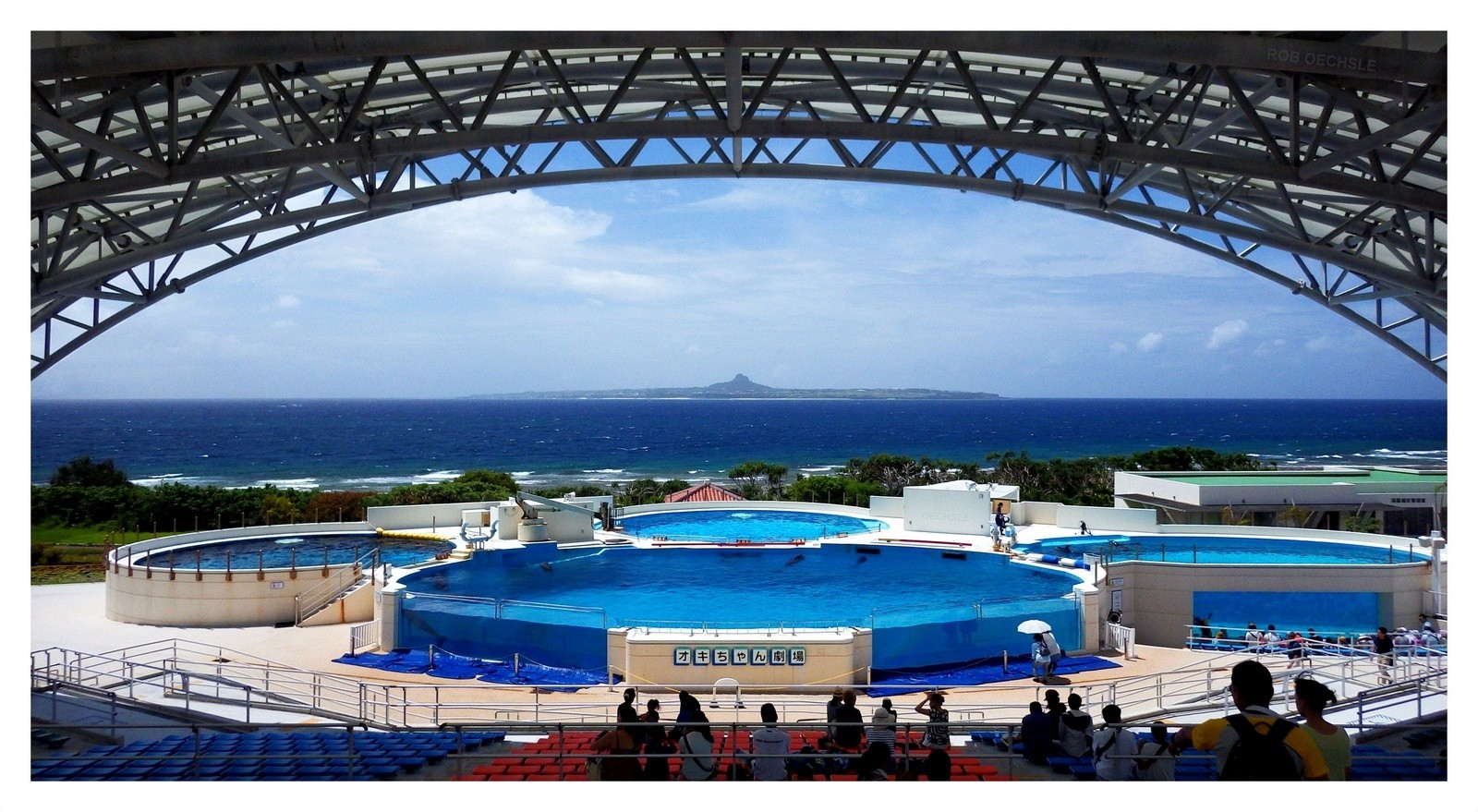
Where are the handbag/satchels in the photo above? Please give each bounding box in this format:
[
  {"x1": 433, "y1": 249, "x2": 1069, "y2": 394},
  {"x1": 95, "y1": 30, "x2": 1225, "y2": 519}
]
[
  {"x1": 1390, "y1": 655, "x2": 1397, "y2": 667},
  {"x1": 1132, "y1": 761, "x2": 1144, "y2": 776},
  {"x1": 1085, "y1": 734, "x2": 1093, "y2": 748}
]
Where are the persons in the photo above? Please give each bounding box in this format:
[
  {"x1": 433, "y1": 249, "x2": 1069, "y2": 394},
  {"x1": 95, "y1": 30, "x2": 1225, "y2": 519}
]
[
  {"x1": 1092, "y1": 705, "x2": 1138, "y2": 780},
  {"x1": 643, "y1": 700, "x2": 660, "y2": 722},
  {"x1": 1080, "y1": 518, "x2": 1091, "y2": 535},
  {"x1": 802, "y1": 746, "x2": 859, "y2": 774},
  {"x1": 734, "y1": 703, "x2": 802, "y2": 780},
  {"x1": 1368, "y1": 627, "x2": 1397, "y2": 685},
  {"x1": 616, "y1": 689, "x2": 644, "y2": 729},
  {"x1": 925, "y1": 751, "x2": 952, "y2": 780},
  {"x1": 1020, "y1": 700, "x2": 1054, "y2": 765},
  {"x1": 669, "y1": 691, "x2": 701, "y2": 738},
  {"x1": 1193, "y1": 608, "x2": 1449, "y2": 652},
  {"x1": 1042, "y1": 690, "x2": 1065, "y2": 738},
  {"x1": 1138, "y1": 722, "x2": 1175, "y2": 780},
  {"x1": 591, "y1": 707, "x2": 645, "y2": 780},
  {"x1": 1061, "y1": 693, "x2": 1095, "y2": 759},
  {"x1": 866, "y1": 708, "x2": 898, "y2": 773},
  {"x1": 1030, "y1": 634, "x2": 1050, "y2": 683},
  {"x1": 882, "y1": 699, "x2": 899, "y2": 731},
  {"x1": 1171, "y1": 660, "x2": 1330, "y2": 780},
  {"x1": 677, "y1": 711, "x2": 719, "y2": 781},
  {"x1": 827, "y1": 686, "x2": 845, "y2": 737},
  {"x1": 1043, "y1": 631, "x2": 1061, "y2": 676},
  {"x1": 1292, "y1": 670, "x2": 1353, "y2": 781},
  {"x1": 835, "y1": 692, "x2": 866, "y2": 746},
  {"x1": 645, "y1": 725, "x2": 677, "y2": 780},
  {"x1": 1280, "y1": 630, "x2": 1304, "y2": 669},
  {"x1": 857, "y1": 741, "x2": 913, "y2": 781},
  {"x1": 995, "y1": 503, "x2": 1006, "y2": 536},
  {"x1": 916, "y1": 690, "x2": 951, "y2": 750}
]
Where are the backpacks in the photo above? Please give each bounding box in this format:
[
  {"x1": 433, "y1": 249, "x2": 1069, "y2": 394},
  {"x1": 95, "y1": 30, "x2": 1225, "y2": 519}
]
[
  {"x1": 584, "y1": 756, "x2": 602, "y2": 781},
  {"x1": 1037, "y1": 639, "x2": 1051, "y2": 657},
  {"x1": 1220, "y1": 714, "x2": 1305, "y2": 781}
]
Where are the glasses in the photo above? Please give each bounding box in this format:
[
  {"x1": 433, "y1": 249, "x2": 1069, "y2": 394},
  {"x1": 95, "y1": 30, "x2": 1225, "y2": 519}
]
[{"x1": 1226, "y1": 685, "x2": 1233, "y2": 692}]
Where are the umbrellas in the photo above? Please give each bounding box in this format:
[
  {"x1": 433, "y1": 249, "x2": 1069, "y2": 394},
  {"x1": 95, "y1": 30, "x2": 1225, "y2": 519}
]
[{"x1": 1018, "y1": 619, "x2": 1052, "y2": 634}]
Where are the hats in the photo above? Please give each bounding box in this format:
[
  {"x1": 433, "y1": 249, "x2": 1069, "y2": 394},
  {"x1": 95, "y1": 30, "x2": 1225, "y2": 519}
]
[
  {"x1": 1249, "y1": 623, "x2": 1254, "y2": 626},
  {"x1": 871, "y1": 708, "x2": 896, "y2": 726},
  {"x1": 1399, "y1": 627, "x2": 1407, "y2": 632},
  {"x1": 883, "y1": 697, "x2": 891, "y2": 707},
  {"x1": 1215, "y1": 631, "x2": 1226, "y2": 638},
  {"x1": 761, "y1": 703, "x2": 777, "y2": 724},
  {"x1": 1294, "y1": 635, "x2": 1301, "y2": 643},
  {"x1": 830, "y1": 686, "x2": 844, "y2": 697},
  {"x1": 1044, "y1": 690, "x2": 1059, "y2": 701},
  {"x1": 1338, "y1": 636, "x2": 1348, "y2": 644}
]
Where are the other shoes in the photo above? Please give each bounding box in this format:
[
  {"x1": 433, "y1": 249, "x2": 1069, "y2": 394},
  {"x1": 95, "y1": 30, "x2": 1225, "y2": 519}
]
[
  {"x1": 1034, "y1": 679, "x2": 1039, "y2": 682},
  {"x1": 1388, "y1": 680, "x2": 1393, "y2": 684},
  {"x1": 1047, "y1": 672, "x2": 1055, "y2": 677},
  {"x1": 1042, "y1": 680, "x2": 1048, "y2": 684}
]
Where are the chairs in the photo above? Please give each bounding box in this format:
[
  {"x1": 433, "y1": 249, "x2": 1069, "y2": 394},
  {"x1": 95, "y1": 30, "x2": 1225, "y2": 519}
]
[{"x1": 32, "y1": 628, "x2": 1447, "y2": 782}]
